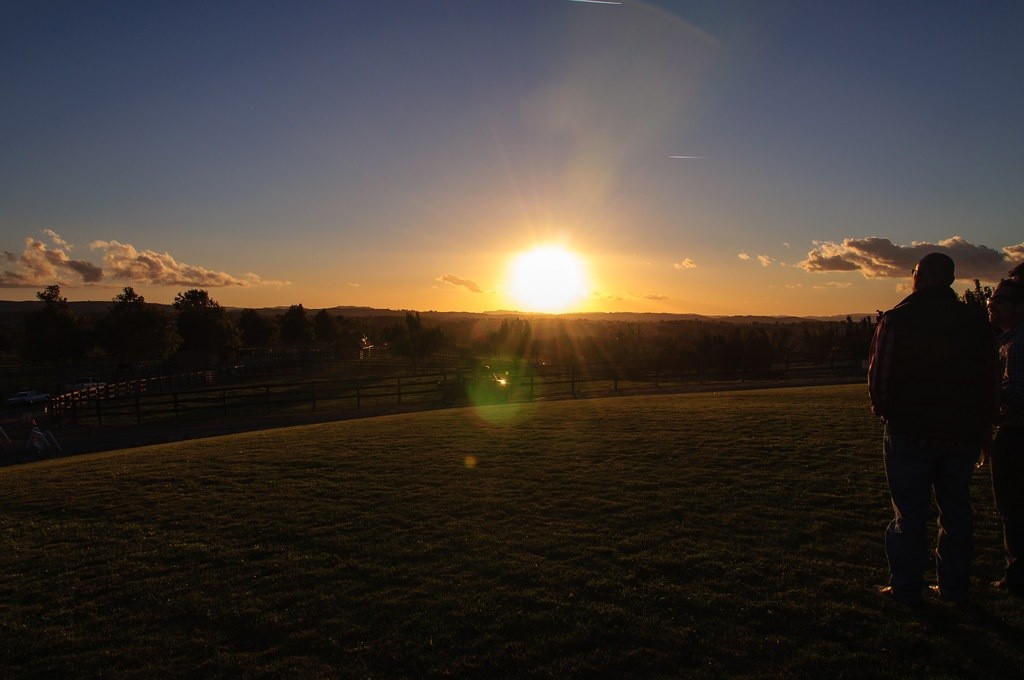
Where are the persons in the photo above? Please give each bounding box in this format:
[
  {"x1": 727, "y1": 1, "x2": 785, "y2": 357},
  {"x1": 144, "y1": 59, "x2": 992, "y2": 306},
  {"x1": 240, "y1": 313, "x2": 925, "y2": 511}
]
[
  {"x1": 1009, "y1": 262, "x2": 1024, "y2": 280},
  {"x1": 866, "y1": 250, "x2": 1002, "y2": 617},
  {"x1": 986, "y1": 278, "x2": 1024, "y2": 598}
]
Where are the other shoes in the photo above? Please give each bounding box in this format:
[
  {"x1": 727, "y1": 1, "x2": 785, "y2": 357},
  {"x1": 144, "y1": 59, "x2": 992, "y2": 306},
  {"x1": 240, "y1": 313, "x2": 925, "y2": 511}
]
[
  {"x1": 881, "y1": 587, "x2": 895, "y2": 595},
  {"x1": 929, "y1": 585, "x2": 942, "y2": 597},
  {"x1": 991, "y1": 578, "x2": 1007, "y2": 589}
]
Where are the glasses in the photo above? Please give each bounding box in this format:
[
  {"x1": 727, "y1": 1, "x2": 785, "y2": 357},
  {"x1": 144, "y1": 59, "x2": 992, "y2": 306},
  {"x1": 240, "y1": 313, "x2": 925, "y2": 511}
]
[
  {"x1": 911, "y1": 268, "x2": 920, "y2": 275},
  {"x1": 985, "y1": 295, "x2": 1011, "y2": 308}
]
